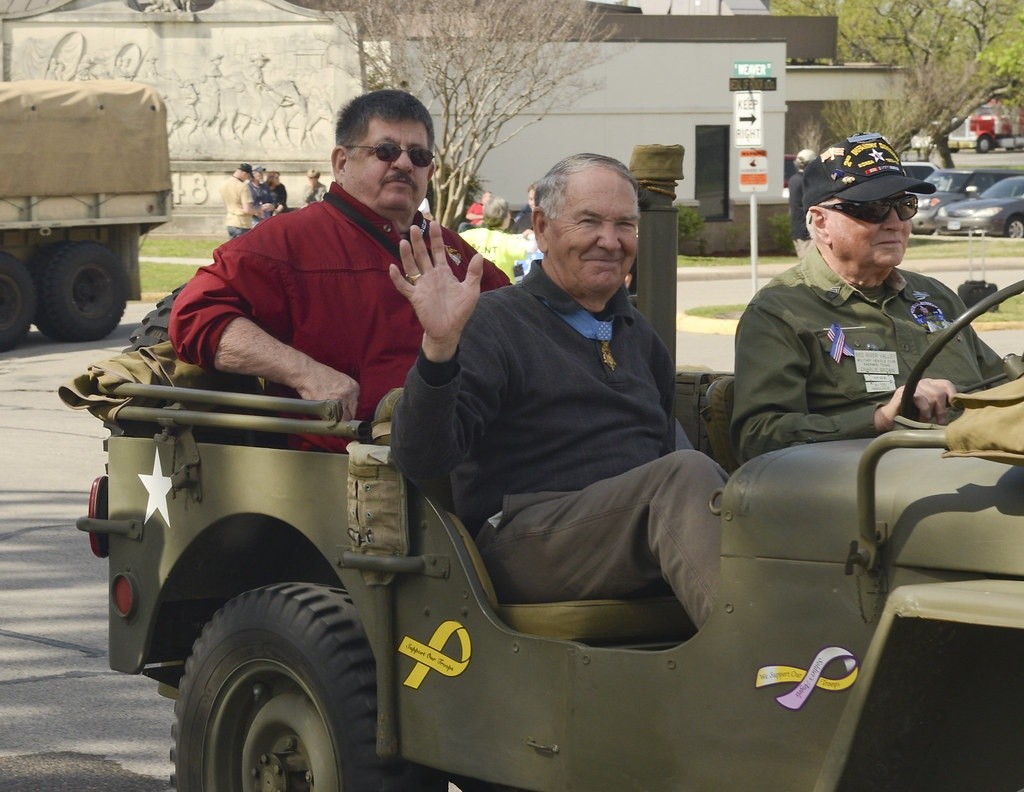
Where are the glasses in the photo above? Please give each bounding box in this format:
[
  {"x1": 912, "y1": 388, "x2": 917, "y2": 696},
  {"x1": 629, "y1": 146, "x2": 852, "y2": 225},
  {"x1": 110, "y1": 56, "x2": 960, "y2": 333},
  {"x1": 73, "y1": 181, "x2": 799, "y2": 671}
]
[
  {"x1": 818, "y1": 194, "x2": 919, "y2": 225},
  {"x1": 344, "y1": 143, "x2": 435, "y2": 167}
]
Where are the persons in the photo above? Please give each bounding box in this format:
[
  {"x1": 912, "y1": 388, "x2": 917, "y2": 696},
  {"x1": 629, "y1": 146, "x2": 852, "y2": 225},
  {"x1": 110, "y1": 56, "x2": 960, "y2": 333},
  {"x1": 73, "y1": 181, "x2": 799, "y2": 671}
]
[
  {"x1": 224, "y1": 164, "x2": 287, "y2": 239},
  {"x1": 625, "y1": 255, "x2": 637, "y2": 295},
  {"x1": 303, "y1": 169, "x2": 326, "y2": 206},
  {"x1": 788, "y1": 150, "x2": 816, "y2": 256},
  {"x1": 387, "y1": 154, "x2": 731, "y2": 630},
  {"x1": 514, "y1": 184, "x2": 539, "y2": 234},
  {"x1": 459, "y1": 197, "x2": 537, "y2": 284},
  {"x1": 457, "y1": 191, "x2": 492, "y2": 233},
  {"x1": 170, "y1": 90, "x2": 511, "y2": 453},
  {"x1": 733, "y1": 133, "x2": 1010, "y2": 464}
]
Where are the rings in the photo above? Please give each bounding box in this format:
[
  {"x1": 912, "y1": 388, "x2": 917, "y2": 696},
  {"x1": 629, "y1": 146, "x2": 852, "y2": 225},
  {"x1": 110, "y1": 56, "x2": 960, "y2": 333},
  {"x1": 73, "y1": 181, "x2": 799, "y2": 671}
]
[{"x1": 407, "y1": 273, "x2": 422, "y2": 284}]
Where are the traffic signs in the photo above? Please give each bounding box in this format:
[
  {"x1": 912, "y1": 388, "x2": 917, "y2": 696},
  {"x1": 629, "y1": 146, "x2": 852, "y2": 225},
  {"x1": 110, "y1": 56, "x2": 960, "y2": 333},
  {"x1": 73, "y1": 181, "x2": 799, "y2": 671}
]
[{"x1": 733, "y1": 91, "x2": 763, "y2": 148}]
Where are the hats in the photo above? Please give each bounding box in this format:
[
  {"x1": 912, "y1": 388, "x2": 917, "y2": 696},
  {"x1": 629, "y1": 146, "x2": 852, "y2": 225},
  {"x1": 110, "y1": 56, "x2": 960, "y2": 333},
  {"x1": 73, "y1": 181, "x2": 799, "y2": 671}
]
[
  {"x1": 800, "y1": 133, "x2": 936, "y2": 214},
  {"x1": 238, "y1": 163, "x2": 255, "y2": 179},
  {"x1": 252, "y1": 164, "x2": 266, "y2": 173}
]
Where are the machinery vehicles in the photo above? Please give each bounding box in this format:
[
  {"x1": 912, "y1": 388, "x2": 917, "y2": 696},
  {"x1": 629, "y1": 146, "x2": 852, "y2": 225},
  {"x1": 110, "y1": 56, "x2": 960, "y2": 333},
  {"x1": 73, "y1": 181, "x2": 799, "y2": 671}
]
[
  {"x1": 948, "y1": 97, "x2": 1024, "y2": 154},
  {"x1": 75, "y1": 281, "x2": 1024, "y2": 792}
]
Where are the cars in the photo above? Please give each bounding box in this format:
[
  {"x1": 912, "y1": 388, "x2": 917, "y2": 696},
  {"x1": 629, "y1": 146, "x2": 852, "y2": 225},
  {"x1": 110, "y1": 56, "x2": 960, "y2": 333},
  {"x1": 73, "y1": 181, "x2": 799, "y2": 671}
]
[
  {"x1": 784, "y1": 154, "x2": 796, "y2": 188},
  {"x1": 904, "y1": 168, "x2": 1024, "y2": 235},
  {"x1": 901, "y1": 161, "x2": 940, "y2": 181},
  {"x1": 934, "y1": 176, "x2": 1024, "y2": 239}
]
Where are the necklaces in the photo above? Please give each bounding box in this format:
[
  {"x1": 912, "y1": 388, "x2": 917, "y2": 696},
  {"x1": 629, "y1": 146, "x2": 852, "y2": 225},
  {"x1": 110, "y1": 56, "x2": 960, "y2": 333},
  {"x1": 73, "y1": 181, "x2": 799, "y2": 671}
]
[{"x1": 232, "y1": 175, "x2": 243, "y2": 182}]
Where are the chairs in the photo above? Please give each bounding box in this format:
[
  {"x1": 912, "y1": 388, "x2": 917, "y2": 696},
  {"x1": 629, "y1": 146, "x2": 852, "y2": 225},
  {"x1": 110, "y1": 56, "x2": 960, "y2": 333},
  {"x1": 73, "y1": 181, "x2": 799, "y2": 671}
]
[
  {"x1": 702, "y1": 377, "x2": 739, "y2": 477},
  {"x1": 379, "y1": 382, "x2": 698, "y2": 650}
]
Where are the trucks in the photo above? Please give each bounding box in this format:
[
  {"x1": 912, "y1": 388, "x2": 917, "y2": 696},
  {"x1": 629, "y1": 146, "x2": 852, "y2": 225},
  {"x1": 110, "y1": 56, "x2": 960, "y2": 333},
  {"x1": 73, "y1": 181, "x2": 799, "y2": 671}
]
[{"x1": 0, "y1": 79, "x2": 175, "y2": 349}]
